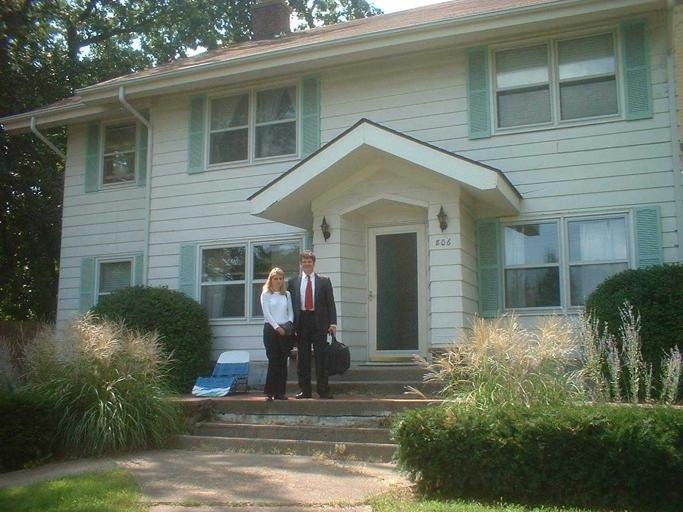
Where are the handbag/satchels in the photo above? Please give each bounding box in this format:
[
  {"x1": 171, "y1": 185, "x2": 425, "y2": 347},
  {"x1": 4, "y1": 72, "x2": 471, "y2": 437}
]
[{"x1": 319, "y1": 332, "x2": 351, "y2": 377}]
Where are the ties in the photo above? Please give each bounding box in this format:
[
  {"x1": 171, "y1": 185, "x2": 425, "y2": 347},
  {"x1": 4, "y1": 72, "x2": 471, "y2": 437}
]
[{"x1": 305, "y1": 275, "x2": 313, "y2": 311}]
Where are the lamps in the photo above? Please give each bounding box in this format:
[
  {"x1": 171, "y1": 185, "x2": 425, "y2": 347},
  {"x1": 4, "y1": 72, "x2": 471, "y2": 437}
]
[
  {"x1": 320, "y1": 216, "x2": 330, "y2": 241},
  {"x1": 436, "y1": 206, "x2": 446, "y2": 231}
]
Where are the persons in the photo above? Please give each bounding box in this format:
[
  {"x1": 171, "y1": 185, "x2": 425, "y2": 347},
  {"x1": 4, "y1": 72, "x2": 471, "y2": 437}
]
[
  {"x1": 260, "y1": 267, "x2": 294, "y2": 399},
  {"x1": 286, "y1": 250, "x2": 337, "y2": 399}
]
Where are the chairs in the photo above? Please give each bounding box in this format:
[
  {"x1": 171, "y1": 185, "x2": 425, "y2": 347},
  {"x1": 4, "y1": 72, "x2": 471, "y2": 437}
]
[{"x1": 191, "y1": 350, "x2": 250, "y2": 397}]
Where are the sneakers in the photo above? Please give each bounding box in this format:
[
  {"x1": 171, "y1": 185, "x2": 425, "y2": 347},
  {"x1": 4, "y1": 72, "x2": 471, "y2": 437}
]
[
  {"x1": 319, "y1": 393, "x2": 333, "y2": 400},
  {"x1": 294, "y1": 391, "x2": 312, "y2": 399},
  {"x1": 263, "y1": 393, "x2": 289, "y2": 401}
]
